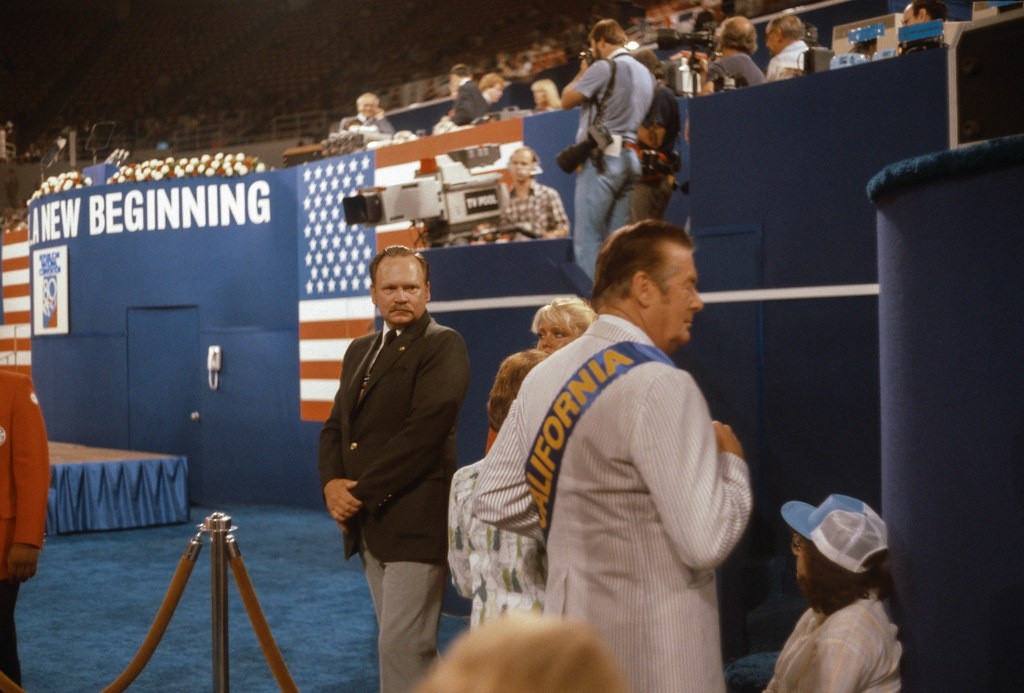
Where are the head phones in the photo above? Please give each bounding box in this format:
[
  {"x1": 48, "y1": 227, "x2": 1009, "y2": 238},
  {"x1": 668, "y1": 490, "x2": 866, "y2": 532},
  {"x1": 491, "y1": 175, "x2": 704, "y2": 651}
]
[{"x1": 506, "y1": 159, "x2": 543, "y2": 176}]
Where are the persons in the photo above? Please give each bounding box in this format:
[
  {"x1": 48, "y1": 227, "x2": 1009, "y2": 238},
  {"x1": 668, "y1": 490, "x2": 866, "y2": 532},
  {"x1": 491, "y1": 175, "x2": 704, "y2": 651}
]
[
  {"x1": 25, "y1": 143, "x2": 40, "y2": 158},
  {"x1": 415, "y1": 617, "x2": 624, "y2": 693},
  {"x1": 448, "y1": 52, "x2": 513, "y2": 127},
  {"x1": 318, "y1": 245, "x2": 471, "y2": 692},
  {"x1": 765, "y1": 14, "x2": 808, "y2": 82},
  {"x1": 561, "y1": 19, "x2": 653, "y2": 288},
  {"x1": 477, "y1": 145, "x2": 570, "y2": 244},
  {"x1": 472, "y1": 219, "x2": 750, "y2": 692},
  {"x1": 764, "y1": 494, "x2": 904, "y2": 693},
  {"x1": 0, "y1": 369, "x2": 49, "y2": 690},
  {"x1": 631, "y1": 50, "x2": 681, "y2": 223},
  {"x1": 531, "y1": 298, "x2": 595, "y2": 354},
  {"x1": 448, "y1": 351, "x2": 551, "y2": 626},
  {"x1": 340, "y1": 92, "x2": 395, "y2": 134},
  {"x1": 531, "y1": 78, "x2": 562, "y2": 111},
  {"x1": 902, "y1": 0, "x2": 948, "y2": 43},
  {"x1": 694, "y1": 16, "x2": 764, "y2": 97},
  {"x1": 693, "y1": 3, "x2": 714, "y2": 32}
]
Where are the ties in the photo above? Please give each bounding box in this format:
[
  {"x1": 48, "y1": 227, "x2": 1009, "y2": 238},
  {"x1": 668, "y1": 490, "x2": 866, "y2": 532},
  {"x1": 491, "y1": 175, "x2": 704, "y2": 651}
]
[{"x1": 369, "y1": 329, "x2": 398, "y2": 376}]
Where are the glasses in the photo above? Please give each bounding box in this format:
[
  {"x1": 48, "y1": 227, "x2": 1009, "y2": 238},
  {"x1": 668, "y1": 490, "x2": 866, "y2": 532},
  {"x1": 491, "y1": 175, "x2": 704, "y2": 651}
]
[
  {"x1": 762, "y1": 30, "x2": 774, "y2": 43},
  {"x1": 900, "y1": 15, "x2": 917, "y2": 27},
  {"x1": 791, "y1": 533, "x2": 808, "y2": 555}
]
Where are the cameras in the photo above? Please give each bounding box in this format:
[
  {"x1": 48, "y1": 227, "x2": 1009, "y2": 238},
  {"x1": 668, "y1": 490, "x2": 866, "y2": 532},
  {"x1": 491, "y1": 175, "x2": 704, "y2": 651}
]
[
  {"x1": 578, "y1": 50, "x2": 593, "y2": 67},
  {"x1": 556, "y1": 124, "x2": 613, "y2": 173},
  {"x1": 658, "y1": 29, "x2": 715, "y2": 50}
]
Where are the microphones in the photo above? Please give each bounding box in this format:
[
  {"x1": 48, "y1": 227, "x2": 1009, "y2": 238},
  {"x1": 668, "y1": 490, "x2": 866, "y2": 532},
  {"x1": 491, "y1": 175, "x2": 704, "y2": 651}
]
[{"x1": 520, "y1": 170, "x2": 530, "y2": 177}]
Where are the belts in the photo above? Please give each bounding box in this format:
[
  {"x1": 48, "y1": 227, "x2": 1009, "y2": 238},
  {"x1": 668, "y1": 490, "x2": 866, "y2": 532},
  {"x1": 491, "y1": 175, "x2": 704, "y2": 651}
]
[{"x1": 622, "y1": 142, "x2": 639, "y2": 152}]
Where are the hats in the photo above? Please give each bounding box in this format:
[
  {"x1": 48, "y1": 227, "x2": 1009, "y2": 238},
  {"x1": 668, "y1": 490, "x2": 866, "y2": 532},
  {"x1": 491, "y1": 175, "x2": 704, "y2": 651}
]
[{"x1": 782, "y1": 493, "x2": 888, "y2": 572}]
